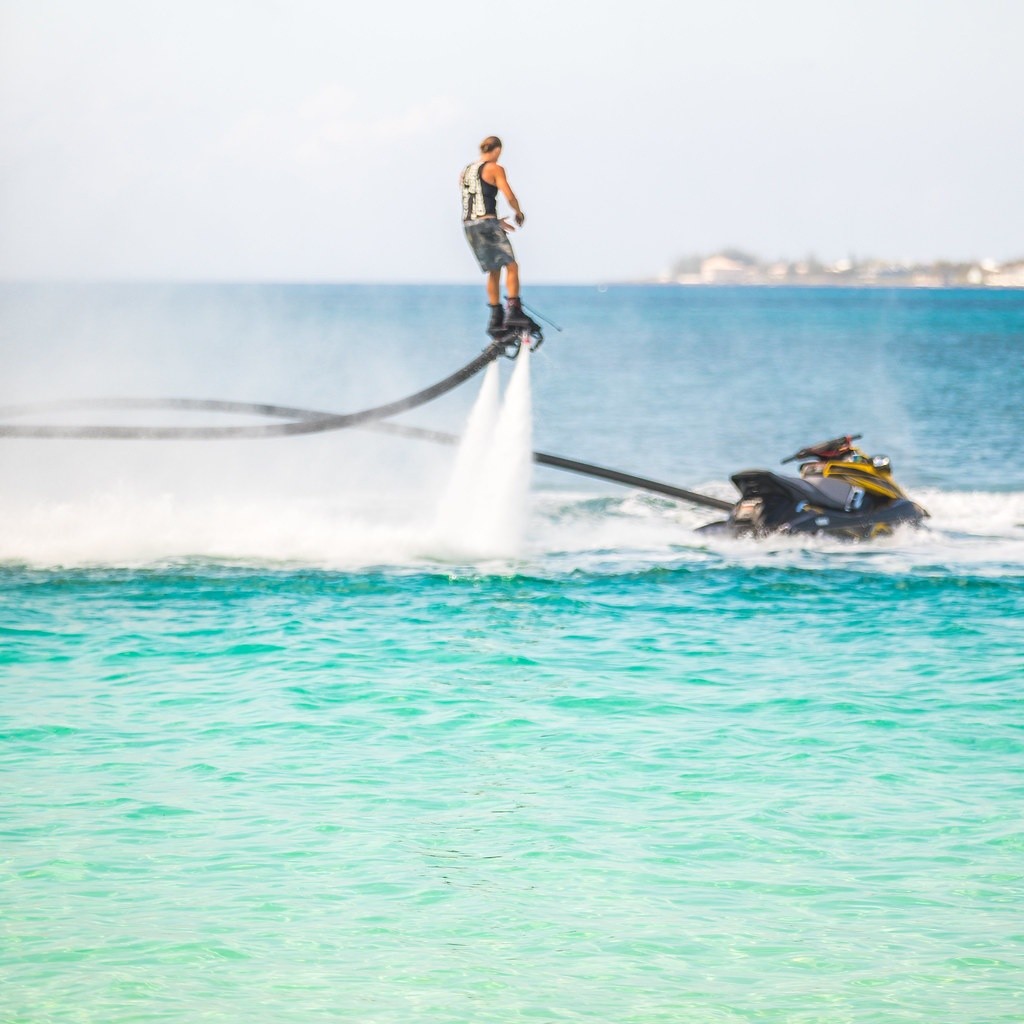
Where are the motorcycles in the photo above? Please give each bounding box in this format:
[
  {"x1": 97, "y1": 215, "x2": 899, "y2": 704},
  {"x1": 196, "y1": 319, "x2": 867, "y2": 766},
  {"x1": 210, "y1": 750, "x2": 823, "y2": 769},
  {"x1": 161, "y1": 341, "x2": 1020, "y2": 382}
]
[{"x1": 697, "y1": 433, "x2": 931, "y2": 541}]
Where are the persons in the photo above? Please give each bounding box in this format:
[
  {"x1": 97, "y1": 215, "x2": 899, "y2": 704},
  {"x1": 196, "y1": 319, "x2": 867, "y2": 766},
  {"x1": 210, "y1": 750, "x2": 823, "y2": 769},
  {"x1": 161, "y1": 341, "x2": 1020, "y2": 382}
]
[{"x1": 458, "y1": 134, "x2": 536, "y2": 331}]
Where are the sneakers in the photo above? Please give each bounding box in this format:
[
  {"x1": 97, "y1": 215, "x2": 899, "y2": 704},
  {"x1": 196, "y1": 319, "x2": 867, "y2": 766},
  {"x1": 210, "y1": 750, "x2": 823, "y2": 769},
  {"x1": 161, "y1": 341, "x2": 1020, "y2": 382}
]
[
  {"x1": 503, "y1": 297, "x2": 532, "y2": 326},
  {"x1": 488, "y1": 304, "x2": 505, "y2": 328}
]
